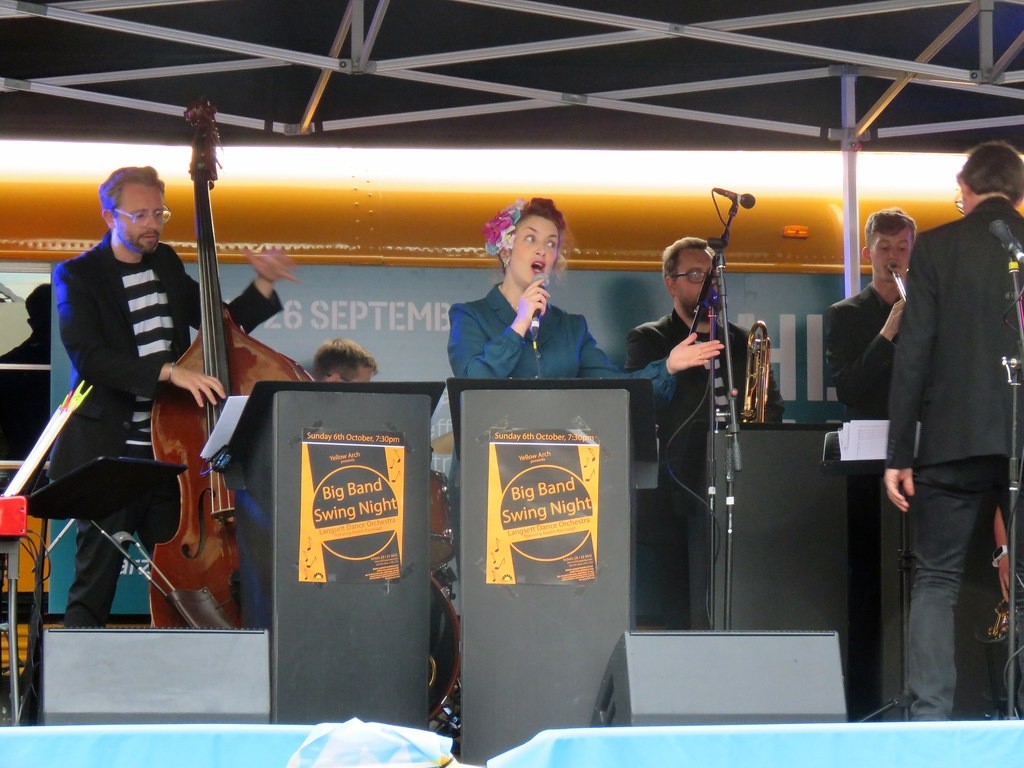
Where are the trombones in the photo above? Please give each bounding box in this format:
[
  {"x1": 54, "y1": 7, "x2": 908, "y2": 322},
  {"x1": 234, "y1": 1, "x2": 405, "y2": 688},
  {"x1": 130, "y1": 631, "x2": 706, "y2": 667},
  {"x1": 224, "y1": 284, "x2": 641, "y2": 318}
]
[{"x1": 742, "y1": 318, "x2": 772, "y2": 424}]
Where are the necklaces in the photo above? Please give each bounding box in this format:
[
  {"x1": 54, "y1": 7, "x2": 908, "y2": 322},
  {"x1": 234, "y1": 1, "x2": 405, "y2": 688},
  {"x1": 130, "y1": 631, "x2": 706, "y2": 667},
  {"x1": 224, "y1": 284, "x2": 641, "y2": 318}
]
[{"x1": 499, "y1": 284, "x2": 519, "y2": 312}]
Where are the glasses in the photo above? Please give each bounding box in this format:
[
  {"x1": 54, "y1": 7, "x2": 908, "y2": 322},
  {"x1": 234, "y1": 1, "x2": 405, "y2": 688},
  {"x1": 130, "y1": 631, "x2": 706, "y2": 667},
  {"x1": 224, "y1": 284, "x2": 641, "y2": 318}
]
[
  {"x1": 673, "y1": 270, "x2": 720, "y2": 285},
  {"x1": 955, "y1": 200, "x2": 964, "y2": 214},
  {"x1": 114, "y1": 203, "x2": 172, "y2": 227}
]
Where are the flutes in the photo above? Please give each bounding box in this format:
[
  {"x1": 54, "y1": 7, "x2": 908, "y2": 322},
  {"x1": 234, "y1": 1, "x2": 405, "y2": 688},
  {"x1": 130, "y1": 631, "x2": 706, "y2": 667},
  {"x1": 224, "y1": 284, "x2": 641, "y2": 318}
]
[{"x1": 887, "y1": 262, "x2": 907, "y2": 302}]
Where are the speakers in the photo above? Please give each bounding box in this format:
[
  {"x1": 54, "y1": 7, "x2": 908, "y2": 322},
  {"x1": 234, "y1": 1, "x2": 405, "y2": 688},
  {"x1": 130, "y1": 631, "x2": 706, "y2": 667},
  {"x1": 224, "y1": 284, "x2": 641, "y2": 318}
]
[
  {"x1": 20, "y1": 629, "x2": 272, "y2": 727},
  {"x1": 590, "y1": 630, "x2": 848, "y2": 727}
]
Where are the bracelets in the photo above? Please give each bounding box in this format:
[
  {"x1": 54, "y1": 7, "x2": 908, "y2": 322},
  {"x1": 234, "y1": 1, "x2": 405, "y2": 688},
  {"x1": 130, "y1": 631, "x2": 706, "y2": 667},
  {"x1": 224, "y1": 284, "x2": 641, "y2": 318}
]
[{"x1": 169, "y1": 362, "x2": 176, "y2": 383}]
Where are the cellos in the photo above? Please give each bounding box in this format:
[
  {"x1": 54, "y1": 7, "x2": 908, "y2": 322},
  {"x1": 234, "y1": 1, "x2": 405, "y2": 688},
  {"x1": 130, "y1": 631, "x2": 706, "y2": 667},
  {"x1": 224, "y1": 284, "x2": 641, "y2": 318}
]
[{"x1": 150, "y1": 90, "x2": 321, "y2": 633}]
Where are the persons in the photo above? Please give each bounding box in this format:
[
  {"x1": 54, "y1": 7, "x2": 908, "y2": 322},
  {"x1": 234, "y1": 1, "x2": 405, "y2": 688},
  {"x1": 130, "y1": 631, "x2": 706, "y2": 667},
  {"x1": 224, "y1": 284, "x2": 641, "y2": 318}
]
[
  {"x1": 822, "y1": 209, "x2": 912, "y2": 705},
  {"x1": 0, "y1": 281, "x2": 54, "y2": 461},
  {"x1": 620, "y1": 234, "x2": 785, "y2": 625},
  {"x1": 46, "y1": 165, "x2": 296, "y2": 628},
  {"x1": 885, "y1": 142, "x2": 1024, "y2": 721},
  {"x1": 446, "y1": 196, "x2": 724, "y2": 402},
  {"x1": 311, "y1": 338, "x2": 377, "y2": 384}
]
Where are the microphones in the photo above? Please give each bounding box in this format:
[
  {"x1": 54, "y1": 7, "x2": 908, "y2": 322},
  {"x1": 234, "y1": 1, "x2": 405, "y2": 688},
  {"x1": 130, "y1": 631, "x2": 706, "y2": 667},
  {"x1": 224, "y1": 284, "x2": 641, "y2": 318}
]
[
  {"x1": 712, "y1": 187, "x2": 755, "y2": 209},
  {"x1": 529, "y1": 273, "x2": 550, "y2": 340},
  {"x1": 989, "y1": 220, "x2": 1024, "y2": 264}
]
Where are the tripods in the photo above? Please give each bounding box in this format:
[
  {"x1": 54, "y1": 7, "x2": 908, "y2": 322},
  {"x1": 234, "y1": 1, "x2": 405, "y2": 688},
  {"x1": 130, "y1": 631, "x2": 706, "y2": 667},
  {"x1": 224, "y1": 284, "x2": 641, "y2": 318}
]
[{"x1": 822, "y1": 429, "x2": 919, "y2": 724}]
